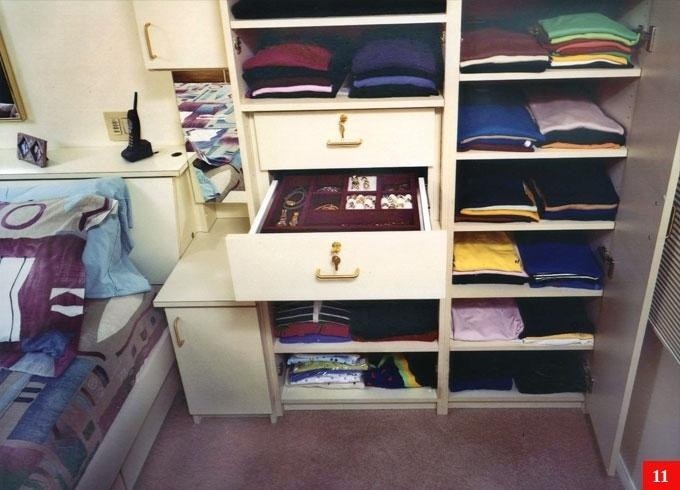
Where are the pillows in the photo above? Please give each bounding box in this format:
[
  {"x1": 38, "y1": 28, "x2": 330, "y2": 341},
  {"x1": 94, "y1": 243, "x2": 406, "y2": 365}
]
[
  {"x1": 0, "y1": 195, "x2": 118, "y2": 379},
  {"x1": 0, "y1": 175, "x2": 152, "y2": 299}
]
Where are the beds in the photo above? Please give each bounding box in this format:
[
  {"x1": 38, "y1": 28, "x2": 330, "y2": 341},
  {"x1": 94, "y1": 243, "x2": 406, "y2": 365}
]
[{"x1": 0, "y1": 141, "x2": 194, "y2": 489}]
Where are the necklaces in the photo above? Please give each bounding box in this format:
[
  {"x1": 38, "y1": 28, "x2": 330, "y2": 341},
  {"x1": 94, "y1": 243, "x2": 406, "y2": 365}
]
[{"x1": 283, "y1": 185, "x2": 307, "y2": 210}]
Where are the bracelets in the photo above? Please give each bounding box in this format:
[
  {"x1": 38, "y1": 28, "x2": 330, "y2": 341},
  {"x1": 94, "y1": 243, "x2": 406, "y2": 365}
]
[
  {"x1": 314, "y1": 203, "x2": 339, "y2": 211},
  {"x1": 316, "y1": 185, "x2": 341, "y2": 193}
]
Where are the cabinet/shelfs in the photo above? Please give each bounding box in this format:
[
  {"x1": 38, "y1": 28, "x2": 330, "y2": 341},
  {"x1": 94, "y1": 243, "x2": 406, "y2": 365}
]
[{"x1": 131, "y1": 0, "x2": 679, "y2": 475}]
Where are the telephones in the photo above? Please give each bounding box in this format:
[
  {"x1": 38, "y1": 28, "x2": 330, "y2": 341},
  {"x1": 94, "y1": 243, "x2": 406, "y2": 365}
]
[{"x1": 121, "y1": 92, "x2": 153, "y2": 162}]
